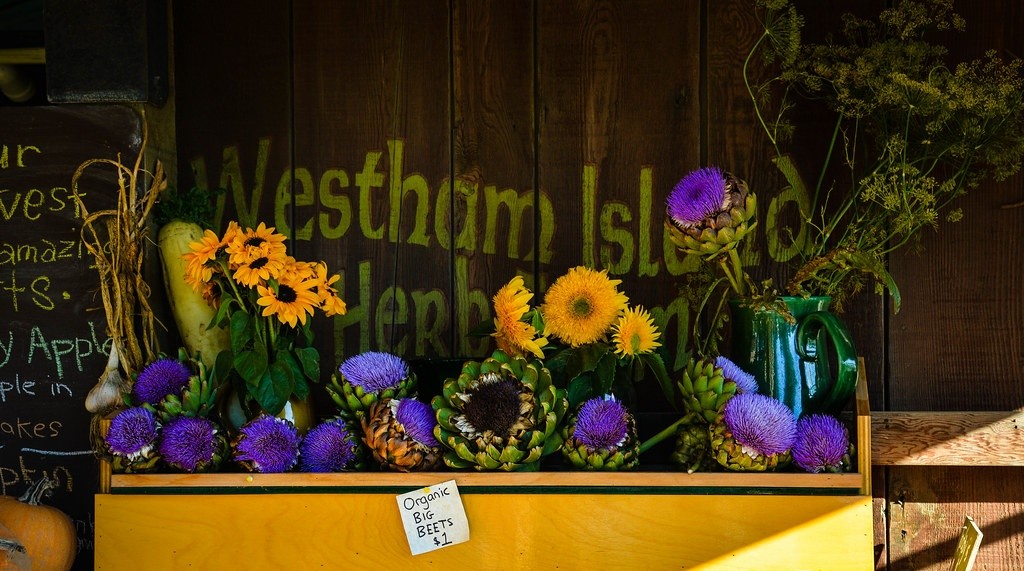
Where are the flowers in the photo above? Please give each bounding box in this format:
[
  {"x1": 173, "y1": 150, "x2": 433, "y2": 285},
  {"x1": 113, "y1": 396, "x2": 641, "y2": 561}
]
[{"x1": 69, "y1": 0, "x2": 1024, "y2": 480}]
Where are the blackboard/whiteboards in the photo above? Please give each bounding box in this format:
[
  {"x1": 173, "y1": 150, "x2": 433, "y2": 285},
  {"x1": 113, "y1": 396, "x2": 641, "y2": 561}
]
[{"x1": 0, "y1": 101, "x2": 148, "y2": 571}]
